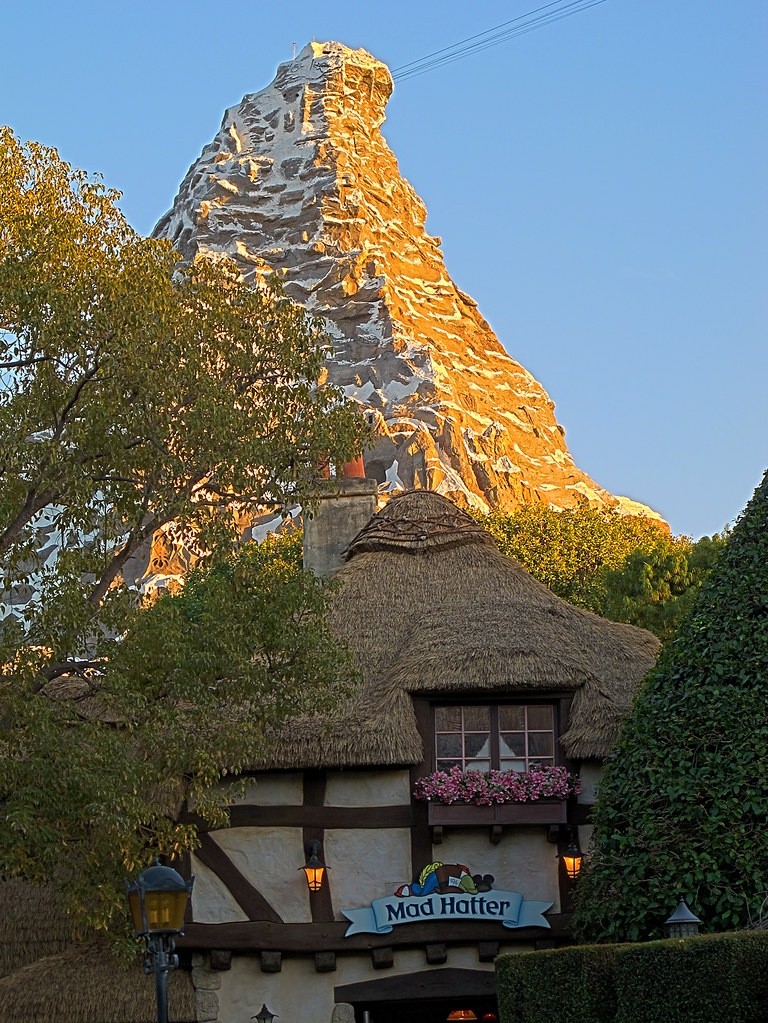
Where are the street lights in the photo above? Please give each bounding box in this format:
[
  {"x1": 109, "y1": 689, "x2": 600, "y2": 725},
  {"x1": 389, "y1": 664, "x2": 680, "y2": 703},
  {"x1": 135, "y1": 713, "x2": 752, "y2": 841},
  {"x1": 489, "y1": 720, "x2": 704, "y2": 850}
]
[{"x1": 125, "y1": 856, "x2": 195, "y2": 1023}]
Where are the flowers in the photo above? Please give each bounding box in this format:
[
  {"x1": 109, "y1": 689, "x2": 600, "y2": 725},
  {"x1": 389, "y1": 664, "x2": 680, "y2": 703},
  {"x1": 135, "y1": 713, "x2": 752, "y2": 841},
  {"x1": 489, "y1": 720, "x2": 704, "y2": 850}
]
[{"x1": 413, "y1": 762, "x2": 583, "y2": 807}]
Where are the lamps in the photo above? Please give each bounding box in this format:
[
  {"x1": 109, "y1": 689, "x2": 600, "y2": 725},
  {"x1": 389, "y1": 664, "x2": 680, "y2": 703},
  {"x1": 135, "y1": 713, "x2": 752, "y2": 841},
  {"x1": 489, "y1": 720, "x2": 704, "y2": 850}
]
[
  {"x1": 483, "y1": 1014, "x2": 496, "y2": 1019},
  {"x1": 447, "y1": 1009, "x2": 477, "y2": 1021},
  {"x1": 556, "y1": 829, "x2": 584, "y2": 880},
  {"x1": 297, "y1": 839, "x2": 331, "y2": 896}
]
